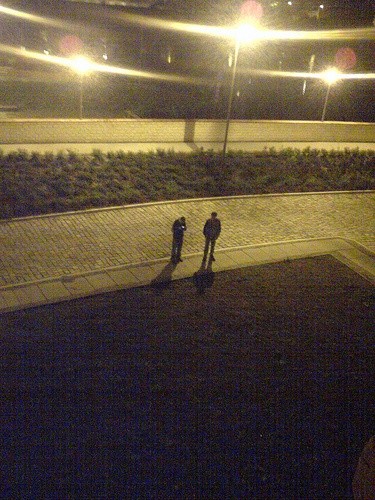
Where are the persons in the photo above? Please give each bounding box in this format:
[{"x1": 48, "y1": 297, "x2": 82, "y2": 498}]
[
  {"x1": 171, "y1": 216, "x2": 187, "y2": 263},
  {"x1": 203, "y1": 212, "x2": 222, "y2": 262}
]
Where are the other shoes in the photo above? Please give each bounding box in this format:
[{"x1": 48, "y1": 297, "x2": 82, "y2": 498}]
[
  {"x1": 171, "y1": 258, "x2": 177, "y2": 264},
  {"x1": 203, "y1": 255, "x2": 208, "y2": 260},
  {"x1": 177, "y1": 259, "x2": 182, "y2": 262},
  {"x1": 210, "y1": 255, "x2": 215, "y2": 261}
]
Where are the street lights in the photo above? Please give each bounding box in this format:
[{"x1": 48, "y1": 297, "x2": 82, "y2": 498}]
[{"x1": 216, "y1": 18, "x2": 254, "y2": 156}]
